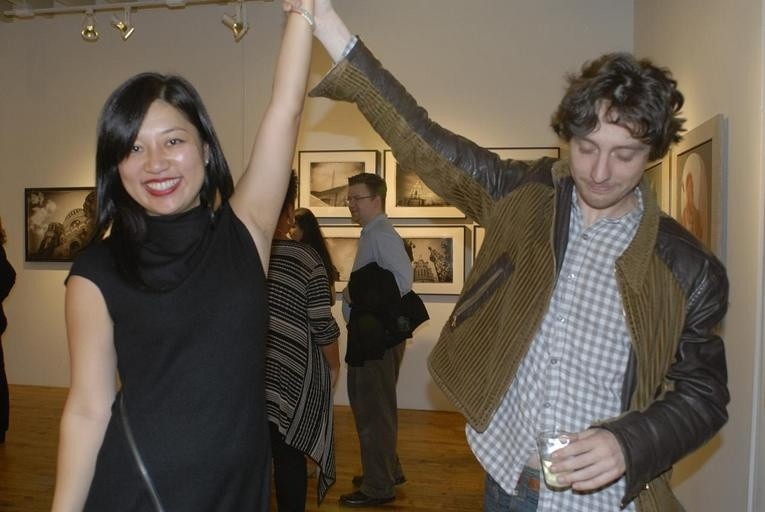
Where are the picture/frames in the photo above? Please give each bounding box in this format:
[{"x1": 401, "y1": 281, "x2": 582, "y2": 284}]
[
  {"x1": 393, "y1": 224, "x2": 466, "y2": 296},
  {"x1": 641, "y1": 148, "x2": 670, "y2": 217},
  {"x1": 472, "y1": 225, "x2": 485, "y2": 265},
  {"x1": 298, "y1": 149, "x2": 378, "y2": 219},
  {"x1": 383, "y1": 148, "x2": 467, "y2": 219},
  {"x1": 669, "y1": 113, "x2": 722, "y2": 261},
  {"x1": 24, "y1": 186, "x2": 97, "y2": 262},
  {"x1": 318, "y1": 224, "x2": 363, "y2": 293},
  {"x1": 471, "y1": 146, "x2": 561, "y2": 162}
]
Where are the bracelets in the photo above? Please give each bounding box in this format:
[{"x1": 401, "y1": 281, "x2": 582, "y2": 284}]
[{"x1": 287, "y1": 6, "x2": 315, "y2": 30}]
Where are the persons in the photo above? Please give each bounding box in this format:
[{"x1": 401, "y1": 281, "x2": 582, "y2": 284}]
[
  {"x1": 50, "y1": 0, "x2": 315, "y2": 512},
  {"x1": 1, "y1": 216, "x2": 16, "y2": 447},
  {"x1": 261, "y1": 165, "x2": 341, "y2": 512},
  {"x1": 282, "y1": 0, "x2": 729, "y2": 512},
  {"x1": 289, "y1": 206, "x2": 338, "y2": 309},
  {"x1": 339, "y1": 172, "x2": 414, "y2": 508}
]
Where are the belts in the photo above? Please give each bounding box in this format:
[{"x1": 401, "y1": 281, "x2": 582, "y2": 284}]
[{"x1": 520, "y1": 476, "x2": 539, "y2": 493}]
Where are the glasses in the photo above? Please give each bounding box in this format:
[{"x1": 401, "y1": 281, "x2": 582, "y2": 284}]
[{"x1": 345, "y1": 195, "x2": 366, "y2": 202}]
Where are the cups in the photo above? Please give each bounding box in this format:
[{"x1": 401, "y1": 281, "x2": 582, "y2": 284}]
[{"x1": 540, "y1": 432, "x2": 581, "y2": 491}]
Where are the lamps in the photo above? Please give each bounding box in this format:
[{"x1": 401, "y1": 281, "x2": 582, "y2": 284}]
[{"x1": 0, "y1": 0, "x2": 250, "y2": 42}]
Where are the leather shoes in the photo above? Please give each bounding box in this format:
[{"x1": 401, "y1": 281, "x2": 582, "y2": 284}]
[
  {"x1": 339, "y1": 492, "x2": 396, "y2": 506},
  {"x1": 352, "y1": 476, "x2": 407, "y2": 487}
]
